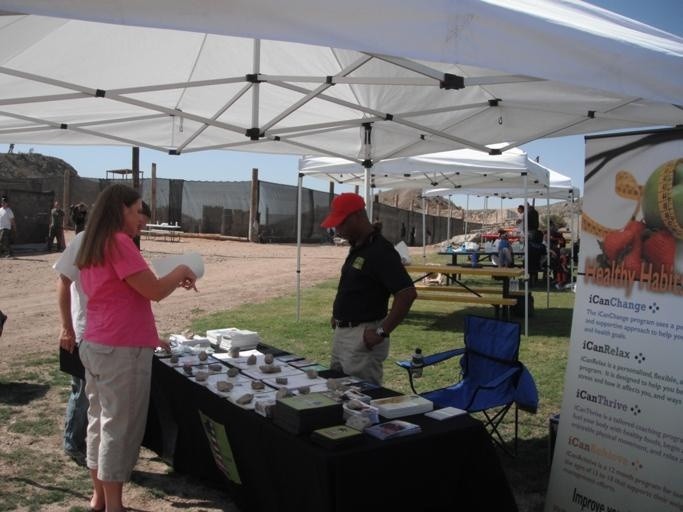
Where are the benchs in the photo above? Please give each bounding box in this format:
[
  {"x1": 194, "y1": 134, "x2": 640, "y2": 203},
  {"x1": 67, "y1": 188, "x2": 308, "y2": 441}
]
[{"x1": 414, "y1": 285, "x2": 525, "y2": 321}]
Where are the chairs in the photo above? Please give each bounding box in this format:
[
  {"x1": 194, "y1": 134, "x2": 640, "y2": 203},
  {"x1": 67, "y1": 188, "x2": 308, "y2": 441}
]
[{"x1": 391, "y1": 314, "x2": 538, "y2": 456}]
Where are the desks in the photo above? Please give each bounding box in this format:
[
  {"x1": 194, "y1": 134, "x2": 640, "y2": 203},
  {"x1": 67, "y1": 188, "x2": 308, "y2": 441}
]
[
  {"x1": 438, "y1": 246, "x2": 523, "y2": 264},
  {"x1": 401, "y1": 263, "x2": 523, "y2": 321},
  {"x1": 140, "y1": 341, "x2": 487, "y2": 512}
]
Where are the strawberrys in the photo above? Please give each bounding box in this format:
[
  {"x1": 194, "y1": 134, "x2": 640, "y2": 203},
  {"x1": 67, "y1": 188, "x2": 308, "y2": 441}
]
[{"x1": 604, "y1": 221, "x2": 675, "y2": 279}]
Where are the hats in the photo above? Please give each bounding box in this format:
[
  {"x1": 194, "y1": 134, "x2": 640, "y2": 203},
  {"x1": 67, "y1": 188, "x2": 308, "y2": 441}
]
[{"x1": 320, "y1": 193, "x2": 366, "y2": 229}]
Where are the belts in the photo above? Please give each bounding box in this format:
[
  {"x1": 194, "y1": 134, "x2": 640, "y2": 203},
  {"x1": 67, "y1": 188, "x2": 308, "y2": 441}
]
[{"x1": 336, "y1": 317, "x2": 375, "y2": 328}]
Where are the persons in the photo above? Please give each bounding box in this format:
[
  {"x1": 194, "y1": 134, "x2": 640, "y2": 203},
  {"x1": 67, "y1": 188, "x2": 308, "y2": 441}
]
[
  {"x1": 493, "y1": 202, "x2": 569, "y2": 291},
  {"x1": 72, "y1": 201, "x2": 88, "y2": 235},
  {"x1": 49, "y1": 200, "x2": 151, "y2": 470},
  {"x1": 0, "y1": 196, "x2": 17, "y2": 259},
  {"x1": 408, "y1": 226, "x2": 416, "y2": 245},
  {"x1": 44, "y1": 201, "x2": 65, "y2": 253},
  {"x1": 372, "y1": 217, "x2": 382, "y2": 235},
  {"x1": 318, "y1": 192, "x2": 419, "y2": 387},
  {"x1": 326, "y1": 227, "x2": 342, "y2": 245},
  {"x1": 400, "y1": 222, "x2": 407, "y2": 245},
  {"x1": 71, "y1": 182, "x2": 202, "y2": 511}
]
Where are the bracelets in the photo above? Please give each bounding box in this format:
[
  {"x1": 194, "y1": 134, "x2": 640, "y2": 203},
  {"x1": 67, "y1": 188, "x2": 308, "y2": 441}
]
[{"x1": 375, "y1": 327, "x2": 389, "y2": 338}]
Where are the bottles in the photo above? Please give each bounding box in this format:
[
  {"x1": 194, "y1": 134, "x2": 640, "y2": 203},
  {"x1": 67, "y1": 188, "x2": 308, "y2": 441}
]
[
  {"x1": 471, "y1": 253, "x2": 477, "y2": 269},
  {"x1": 411, "y1": 348, "x2": 424, "y2": 378}
]
